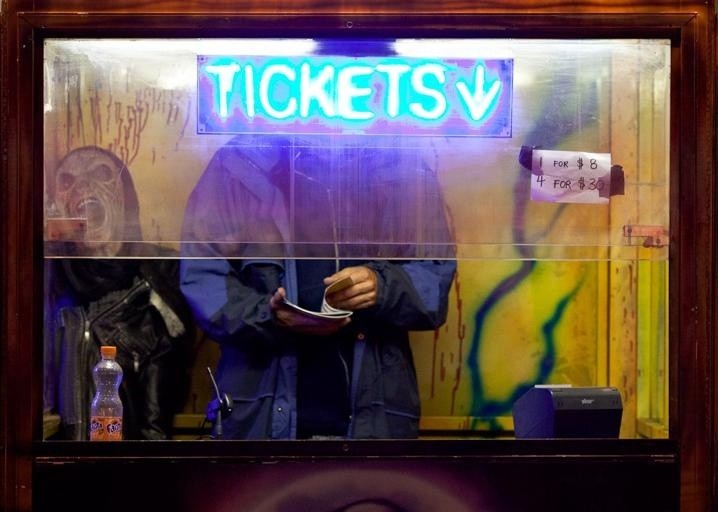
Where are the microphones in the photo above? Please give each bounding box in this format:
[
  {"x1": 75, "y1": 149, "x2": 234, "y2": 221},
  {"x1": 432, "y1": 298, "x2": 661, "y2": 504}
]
[{"x1": 206, "y1": 366, "x2": 223, "y2": 439}]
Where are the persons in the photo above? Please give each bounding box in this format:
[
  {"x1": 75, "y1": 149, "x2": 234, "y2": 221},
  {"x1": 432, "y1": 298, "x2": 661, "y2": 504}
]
[
  {"x1": 180, "y1": 38, "x2": 457, "y2": 440},
  {"x1": 44, "y1": 145, "x2": 199, "y2": 441}
]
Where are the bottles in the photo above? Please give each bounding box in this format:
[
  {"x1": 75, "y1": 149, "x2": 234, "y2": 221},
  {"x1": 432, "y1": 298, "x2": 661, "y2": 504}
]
[{"x1": 89, "y1": 345, "x2": 123, "y2": 441}]
